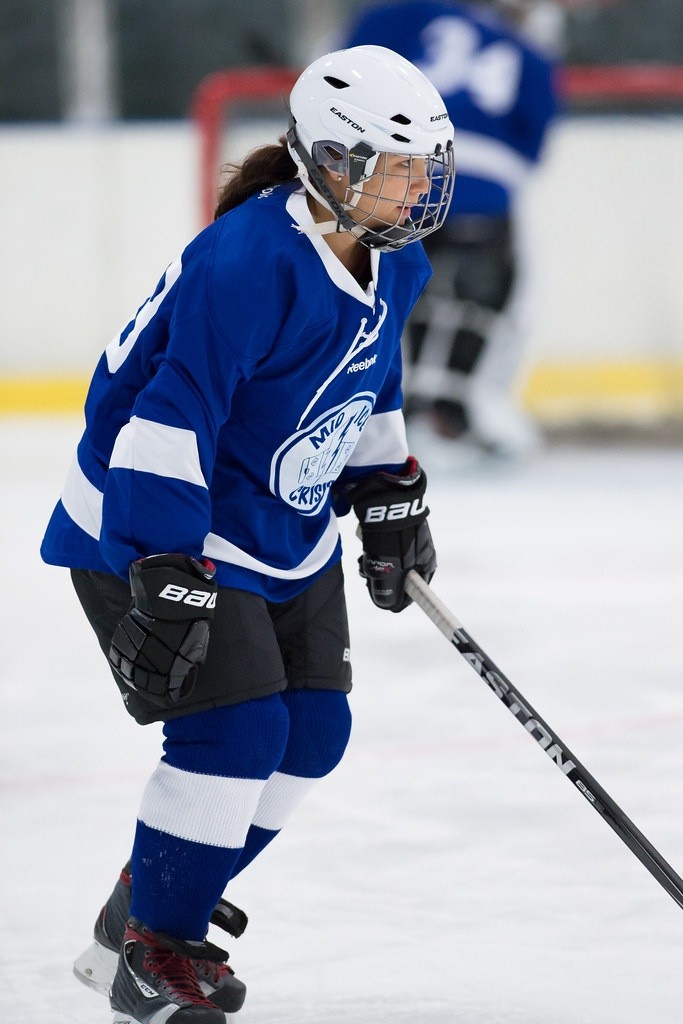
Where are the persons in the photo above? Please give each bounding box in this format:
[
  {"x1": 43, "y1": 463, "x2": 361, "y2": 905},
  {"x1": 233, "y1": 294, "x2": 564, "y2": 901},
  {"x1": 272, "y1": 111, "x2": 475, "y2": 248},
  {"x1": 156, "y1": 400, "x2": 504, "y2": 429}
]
[
  {"x1": 39, "y1": 44, "x2": 458, "y2": 1023},
  {"x1": 332, "y1": 0, "x2": 567, "y2": 486}
]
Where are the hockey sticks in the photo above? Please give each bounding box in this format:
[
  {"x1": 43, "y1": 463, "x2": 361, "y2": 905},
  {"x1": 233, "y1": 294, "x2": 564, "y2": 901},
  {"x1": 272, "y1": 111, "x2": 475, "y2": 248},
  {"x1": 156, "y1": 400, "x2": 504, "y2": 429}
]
[{"x1": 353, "y1": 524, "x2": 683, "y2": 911}]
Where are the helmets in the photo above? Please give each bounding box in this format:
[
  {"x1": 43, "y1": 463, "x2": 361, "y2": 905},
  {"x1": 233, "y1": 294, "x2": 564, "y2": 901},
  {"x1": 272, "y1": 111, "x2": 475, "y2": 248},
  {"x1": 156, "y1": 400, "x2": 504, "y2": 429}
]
[{"x1": 286, "y1": 45, "x2": 454, "y2": 211}]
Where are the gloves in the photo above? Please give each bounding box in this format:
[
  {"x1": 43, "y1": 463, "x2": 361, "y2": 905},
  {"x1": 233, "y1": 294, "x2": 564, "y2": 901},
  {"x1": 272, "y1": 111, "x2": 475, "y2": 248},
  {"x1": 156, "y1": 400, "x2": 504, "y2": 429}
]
[
  {"x1": 108, "y1": 551, "x2": 216, "y2": 704},
  {"x1": 339, "y1": 455, "x2": 437, "y2": 613}
]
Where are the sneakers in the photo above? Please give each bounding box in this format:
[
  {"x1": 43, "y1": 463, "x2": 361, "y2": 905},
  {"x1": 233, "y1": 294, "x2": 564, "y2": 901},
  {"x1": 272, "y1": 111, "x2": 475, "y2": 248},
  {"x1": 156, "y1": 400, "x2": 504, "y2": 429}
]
[
  {"x1": 73, "y1": 871, "x2": 245, "y2": 1012},
  {"x1": 109, "y1": 917, "x2": 229, "y2": 1024}
]
